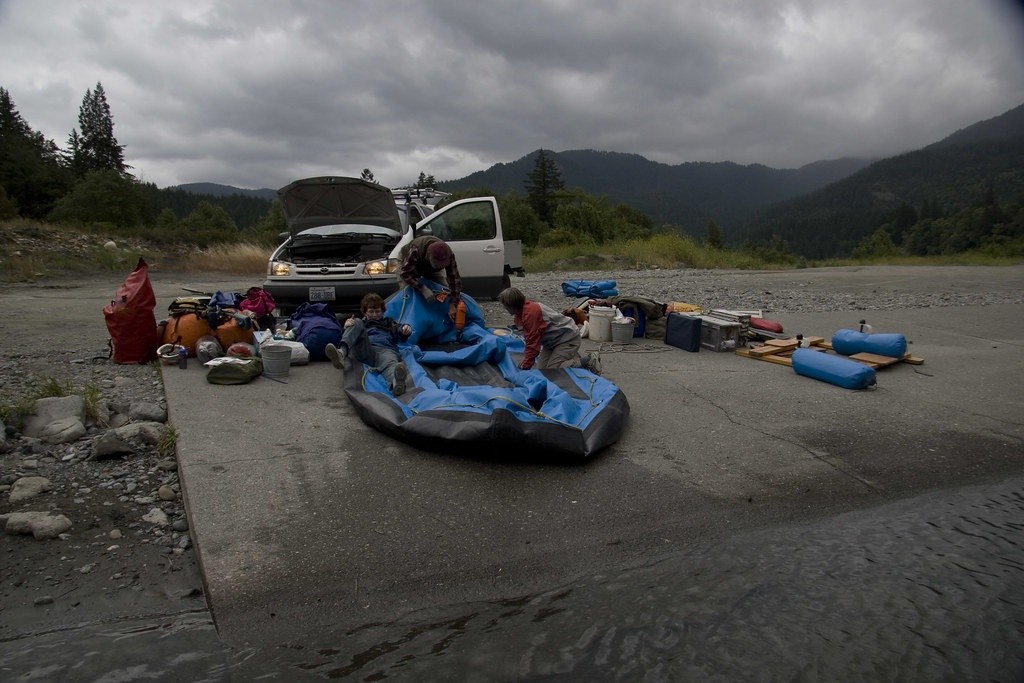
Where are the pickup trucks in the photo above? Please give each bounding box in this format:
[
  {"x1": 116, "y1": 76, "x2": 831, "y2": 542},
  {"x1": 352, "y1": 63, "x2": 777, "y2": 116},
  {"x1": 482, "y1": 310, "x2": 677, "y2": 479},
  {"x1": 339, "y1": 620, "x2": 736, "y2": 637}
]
[{"x1": 490, "y1": 239, "x2": 523, "y2": 301}]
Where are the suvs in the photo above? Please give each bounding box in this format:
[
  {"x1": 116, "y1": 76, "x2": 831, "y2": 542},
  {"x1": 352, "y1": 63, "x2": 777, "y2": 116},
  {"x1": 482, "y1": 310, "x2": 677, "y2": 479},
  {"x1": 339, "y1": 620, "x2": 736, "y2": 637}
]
[{"x1": 262, "y1": 175, "x2": 504, "y2": 316}]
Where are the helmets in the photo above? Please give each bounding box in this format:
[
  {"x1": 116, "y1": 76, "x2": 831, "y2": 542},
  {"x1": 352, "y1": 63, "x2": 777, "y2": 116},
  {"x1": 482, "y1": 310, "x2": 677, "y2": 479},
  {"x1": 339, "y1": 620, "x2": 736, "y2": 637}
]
[{"x1": 156, "y1": 344, "x2": 186, "y2": 365}]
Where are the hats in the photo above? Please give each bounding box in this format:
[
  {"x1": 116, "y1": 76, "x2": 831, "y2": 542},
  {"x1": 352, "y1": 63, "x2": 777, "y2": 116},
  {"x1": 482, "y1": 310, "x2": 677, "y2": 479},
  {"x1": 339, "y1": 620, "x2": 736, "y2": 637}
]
[{"x1": 427, "y1": 241, "x2": 450, "y2": 269}]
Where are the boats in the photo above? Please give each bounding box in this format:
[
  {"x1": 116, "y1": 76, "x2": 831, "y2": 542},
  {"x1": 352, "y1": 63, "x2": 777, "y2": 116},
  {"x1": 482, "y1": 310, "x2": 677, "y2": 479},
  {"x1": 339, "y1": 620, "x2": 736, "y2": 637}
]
[{"x1": 341, "y1": 277, "x2": 632, "y2": 459}]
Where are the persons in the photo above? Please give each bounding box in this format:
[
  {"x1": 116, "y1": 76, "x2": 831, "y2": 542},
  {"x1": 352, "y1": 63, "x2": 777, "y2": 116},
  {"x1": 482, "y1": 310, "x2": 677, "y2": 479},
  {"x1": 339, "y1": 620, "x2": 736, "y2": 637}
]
[
  {"x1": 497, "y1": 287, "x2": 602, "y2": 377},
  {"x1": 396, "y1": 235, "x2": 462, "y2": 324},
  {"x1": 325, "y1": 293, "x2": 412, "y2": 397}
]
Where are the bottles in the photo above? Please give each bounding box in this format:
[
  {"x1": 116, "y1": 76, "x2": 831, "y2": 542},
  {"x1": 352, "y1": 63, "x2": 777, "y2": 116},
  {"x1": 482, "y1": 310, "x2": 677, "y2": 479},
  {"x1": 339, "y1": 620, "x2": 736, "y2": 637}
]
[{"x1": 178, "y1": 349, "x2": 187, "y2": 369}]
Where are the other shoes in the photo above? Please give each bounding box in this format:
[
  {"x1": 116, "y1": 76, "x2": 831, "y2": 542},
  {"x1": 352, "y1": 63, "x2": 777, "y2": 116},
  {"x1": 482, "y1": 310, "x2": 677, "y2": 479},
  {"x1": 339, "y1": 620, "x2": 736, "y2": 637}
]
[
  {"x1": 325, "y1": 343, "x2": 345, "y2": 370},
  {"x1": 393, "y1": 363, "x2": 407, "y2": 395}
]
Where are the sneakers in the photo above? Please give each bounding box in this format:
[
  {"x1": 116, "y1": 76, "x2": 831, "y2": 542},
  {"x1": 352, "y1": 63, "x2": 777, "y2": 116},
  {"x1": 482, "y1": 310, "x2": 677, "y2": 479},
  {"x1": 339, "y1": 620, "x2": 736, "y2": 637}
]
[{"x1": 587, "y1": 352, "x2": 602, "y2": 377}]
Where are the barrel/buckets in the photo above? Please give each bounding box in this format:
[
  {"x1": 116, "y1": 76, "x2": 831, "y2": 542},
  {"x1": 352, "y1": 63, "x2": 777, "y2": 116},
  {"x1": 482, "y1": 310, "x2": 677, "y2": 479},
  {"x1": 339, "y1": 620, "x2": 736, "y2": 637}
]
[
  {"x1": 588, "y1": 304, "x2": 616, "y2": 342},
  {"x1": 260, "y1": 345, "x2": 292, "y2": 378},
  {"x1": 611, "y1": 320, "x2": 636, "y2": 345}
]
